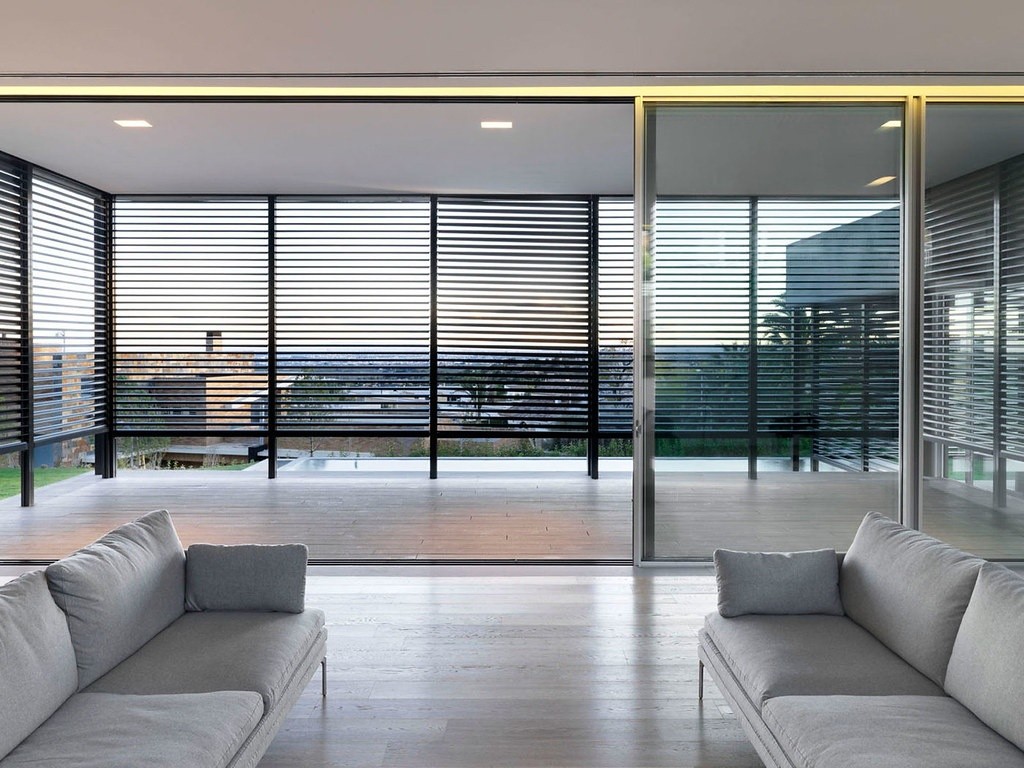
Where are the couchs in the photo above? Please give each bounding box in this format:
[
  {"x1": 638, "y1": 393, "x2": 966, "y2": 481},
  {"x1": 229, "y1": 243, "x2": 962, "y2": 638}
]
[
  {"x1": 0, "y1": 509, "x2": 329, "y2": 768},
  {"x1": 696, "y1": 510, "x2": 1024, "y2": 768}
]
